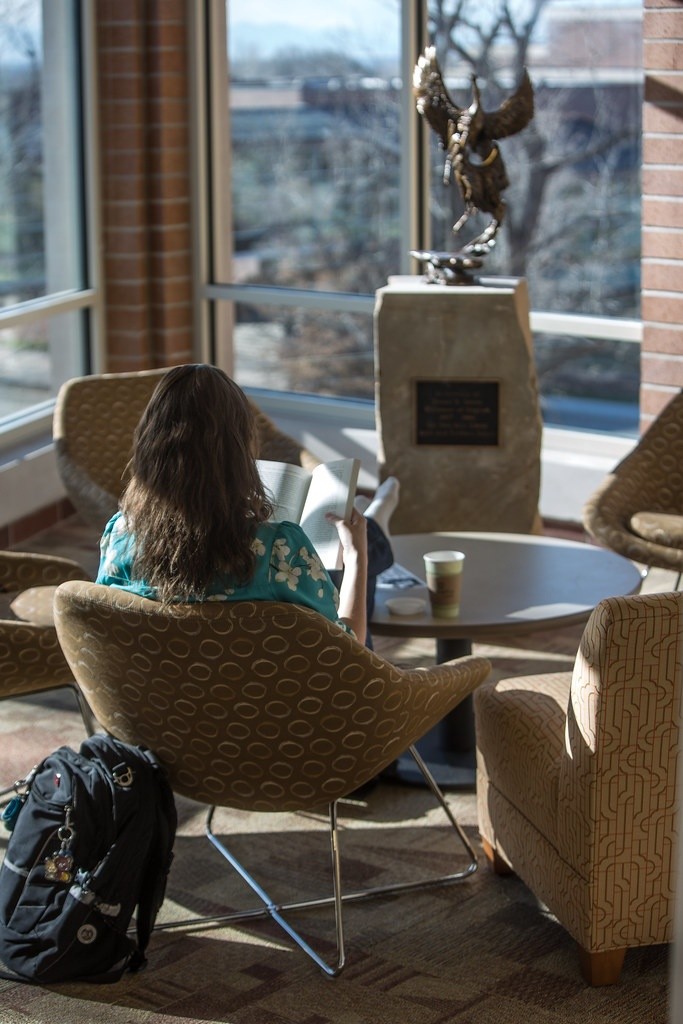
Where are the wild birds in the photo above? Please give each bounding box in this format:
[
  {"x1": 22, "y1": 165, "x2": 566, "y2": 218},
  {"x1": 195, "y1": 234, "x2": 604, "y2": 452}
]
[{"x1": 411, "y1": 32, "x2": 536, "y2": 185}]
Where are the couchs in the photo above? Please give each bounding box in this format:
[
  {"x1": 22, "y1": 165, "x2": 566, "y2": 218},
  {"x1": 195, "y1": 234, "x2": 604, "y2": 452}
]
[{"x1": 473, "y1": 592, "x2": 682, "y2": 987}]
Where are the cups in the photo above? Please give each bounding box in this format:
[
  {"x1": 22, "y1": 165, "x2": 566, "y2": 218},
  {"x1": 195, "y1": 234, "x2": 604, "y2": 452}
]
[{"x1": 423, "y1": 549, "x2": 465, "y2": 618}]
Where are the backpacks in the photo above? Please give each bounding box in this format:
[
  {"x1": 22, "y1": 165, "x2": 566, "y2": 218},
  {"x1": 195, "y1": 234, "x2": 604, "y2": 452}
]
[{"x1": 0, "y1": 735, "x2": 178, "y2": 984}]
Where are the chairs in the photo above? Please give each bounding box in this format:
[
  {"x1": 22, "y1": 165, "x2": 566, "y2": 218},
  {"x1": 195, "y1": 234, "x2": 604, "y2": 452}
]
[
  {"x1": 0, "y1": 549, "x2": 94, "y2": 738},
  {"x1": 51, "y1": 363, "x2": 321, "y2": 533},
  {"x1": 582, "y1": 384, "x2": 683, "y2": 592},
  {"x1": 49, "y1": 579, "x2": 492, "y2": 982}
]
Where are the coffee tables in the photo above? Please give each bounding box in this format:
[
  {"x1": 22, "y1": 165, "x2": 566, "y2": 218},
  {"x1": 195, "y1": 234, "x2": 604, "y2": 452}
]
[{"x1": 370, "y1": 530, "x2": 643, "y2": 787}]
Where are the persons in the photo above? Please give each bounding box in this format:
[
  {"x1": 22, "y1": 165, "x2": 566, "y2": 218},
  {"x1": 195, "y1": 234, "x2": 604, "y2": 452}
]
[{"x1": 96, "y1": 364, "x2": 400, "y2": 656}]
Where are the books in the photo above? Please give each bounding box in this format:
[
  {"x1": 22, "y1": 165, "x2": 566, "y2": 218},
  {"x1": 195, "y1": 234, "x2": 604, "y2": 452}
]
[{"x1": 254, "y1": 456, "x2": 360, "y2": 571}]
